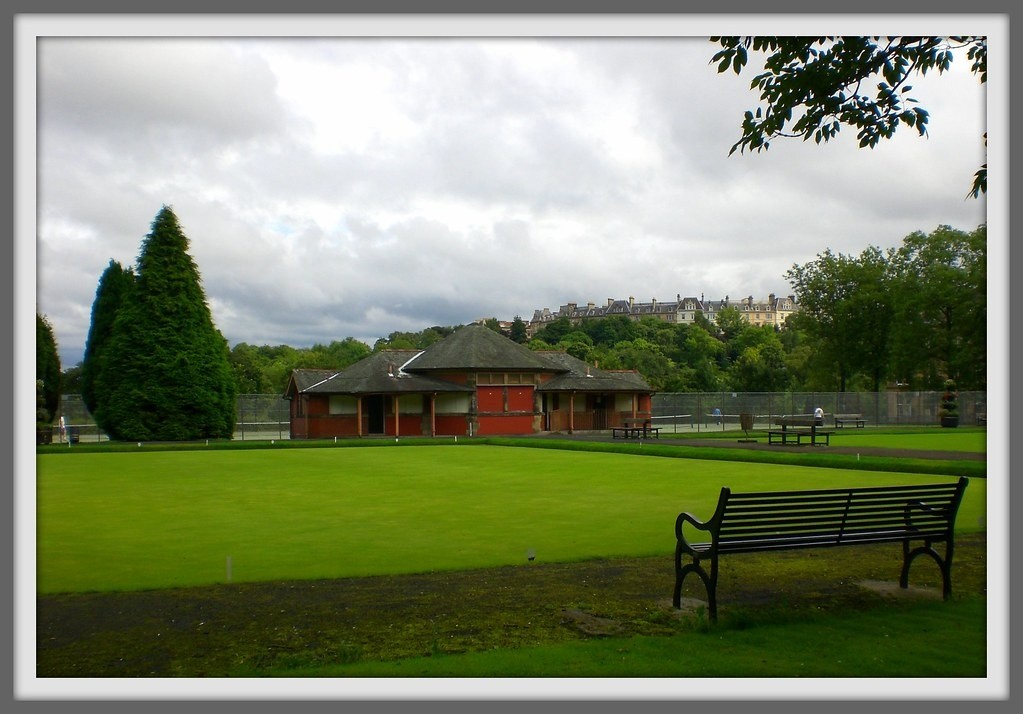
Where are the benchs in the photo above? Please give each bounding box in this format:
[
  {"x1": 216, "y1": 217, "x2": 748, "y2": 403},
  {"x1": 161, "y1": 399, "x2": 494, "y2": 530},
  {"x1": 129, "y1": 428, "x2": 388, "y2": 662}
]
[
  {"x1": 833, "y1": 413, "x2": 868, "y2": 429},
  {"x1": 671, "y1": 474, "x2": 970, "y2": 624},
  {"x1": 763, "y1": 429, "x2": 835, "y2": 446},
  {"x1": 610, "y1": 417, "x2": 662, "y2": 439}
]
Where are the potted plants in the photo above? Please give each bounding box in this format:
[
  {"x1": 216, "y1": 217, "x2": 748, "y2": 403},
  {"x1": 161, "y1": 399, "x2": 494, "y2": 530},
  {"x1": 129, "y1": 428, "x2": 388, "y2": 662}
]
[{"x1": 937, "y1": 378, "x2": 959, "y2": 426}]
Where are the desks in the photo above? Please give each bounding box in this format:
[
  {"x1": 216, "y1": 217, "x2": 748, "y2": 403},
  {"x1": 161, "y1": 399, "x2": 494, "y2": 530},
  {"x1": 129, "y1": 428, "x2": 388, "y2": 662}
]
[{"x1": 775, "y1": 419, "x2": 824, "y2": 446}]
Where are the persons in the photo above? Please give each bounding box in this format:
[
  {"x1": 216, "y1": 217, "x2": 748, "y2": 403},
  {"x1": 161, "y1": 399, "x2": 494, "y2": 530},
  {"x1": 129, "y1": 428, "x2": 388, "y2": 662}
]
[
  {"x1": 814, "y1": 405, "x2": 825, "y2": 424},
  {"x1": 59, "y1": 413, "x2": 67, "y2": 442},
  {"x1": 713, "y1": 407, "x2": 723, "y2": 424}
]
